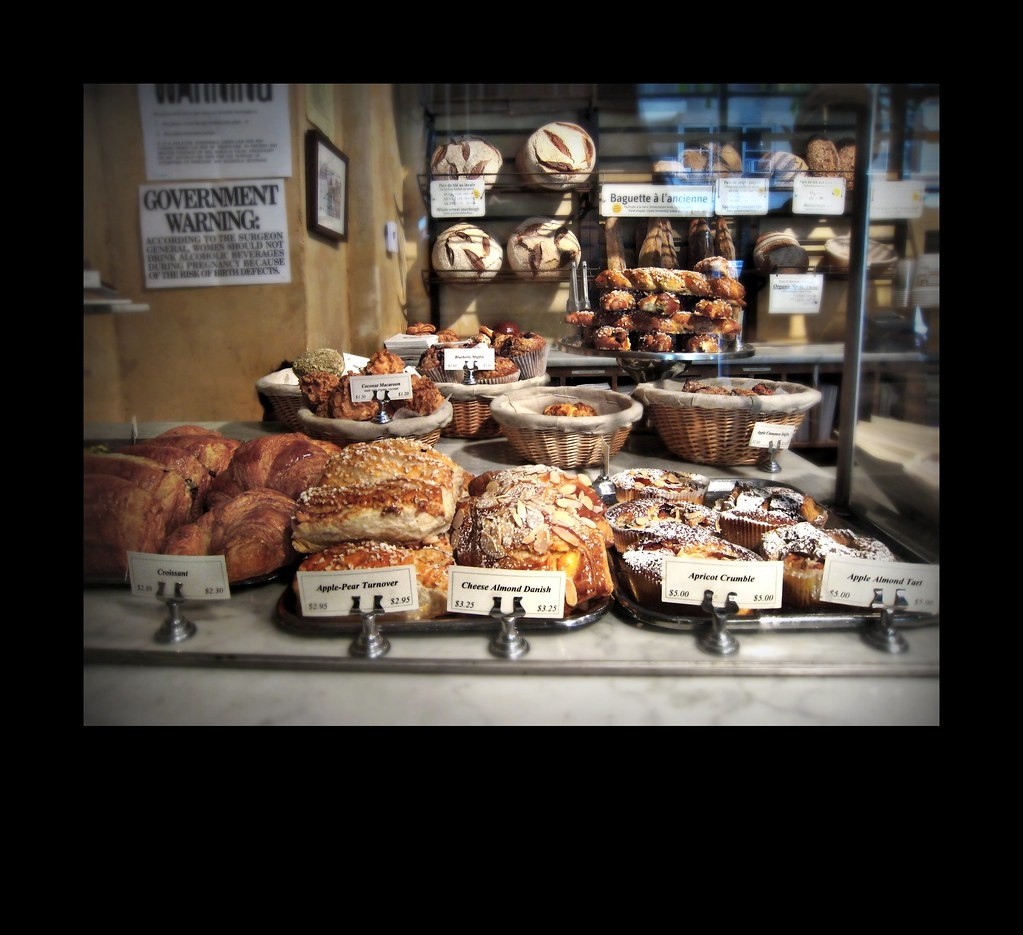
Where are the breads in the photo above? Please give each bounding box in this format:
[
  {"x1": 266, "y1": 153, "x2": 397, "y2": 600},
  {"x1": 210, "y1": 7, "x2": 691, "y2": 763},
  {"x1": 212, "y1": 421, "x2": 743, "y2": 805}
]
[
  {"x1": 406, "y1": 322, "x2": 458, "y2": 343},
  {"x1": 83, "y1": 424, "x2": 340, "y2": 581},
  {"x1": 292, "y1": 338, "x2": 443, "y2": 418},
  {"x1": 564, "y1": 214, "x2": 810, "y2": 354},
  {"x1": 680, "y1": 378, "x2": 780, "y2": 399},
  {"x1": 430, "y1": 121, "x2": 597, "y2": 288},
  {"x1": 292, "y1": 440, "x2": 615, "y2": 620},
  {"x1": 543, "y1": 401, "x2": 601, "y2": 419},
  {"x1": 650, "y1": 135, "x2": 857, "y2": 190}
]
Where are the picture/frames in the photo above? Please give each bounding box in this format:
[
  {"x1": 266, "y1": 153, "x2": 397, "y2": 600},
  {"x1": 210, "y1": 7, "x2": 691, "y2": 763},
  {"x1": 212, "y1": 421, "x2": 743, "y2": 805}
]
[{"x1": 303, "y1": 129, "x2": 350, "y2": 242}]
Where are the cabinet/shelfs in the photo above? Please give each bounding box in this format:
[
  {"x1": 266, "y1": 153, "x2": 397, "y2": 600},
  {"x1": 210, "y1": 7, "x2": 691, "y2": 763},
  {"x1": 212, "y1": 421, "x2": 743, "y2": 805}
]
[
  {"x1": 420, "y1": 169, "x2": 601, "y2": 292},
  {"x1": 547, "y1": 332, "x2": 925, "y2": 452}
]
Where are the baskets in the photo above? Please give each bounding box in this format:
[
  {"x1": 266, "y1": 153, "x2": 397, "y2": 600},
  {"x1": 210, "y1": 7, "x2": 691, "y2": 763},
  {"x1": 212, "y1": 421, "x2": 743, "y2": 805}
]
[
  {"x1": 489, "y1": 386, "x2": 644, "y2": 469},
  {"x1": 635, "y1": 375, "x2": 822, "y2": 467},
  {"x1": 255, "y1": 358, "x2": 361, "y2": 431},
  {"x1": 296, "y1": 393, "x2": 453, "y2": 452},
  {"x1": 431, "y1": 366, "x2": 550, "y2": 438}
]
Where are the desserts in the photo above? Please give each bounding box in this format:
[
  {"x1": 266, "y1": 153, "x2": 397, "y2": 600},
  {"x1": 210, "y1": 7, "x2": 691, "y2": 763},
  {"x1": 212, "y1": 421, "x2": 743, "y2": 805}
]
[
  {"x1": 418, "y1": 326, "x2": 550, "y2": 384},
  {"x1": 605, "y1": 467, "x2": 894, "y2": 608}
]
